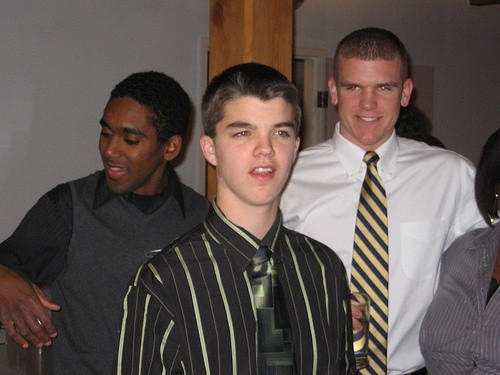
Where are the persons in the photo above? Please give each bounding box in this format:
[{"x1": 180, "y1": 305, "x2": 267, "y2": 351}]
[
  {"x1": 278, "y1": 27, "x2": 489, "y2": 375},
  {"x1": 113, "y1": 61, "x2": 359, "y2": 375},
  {"x1": 419, "y1": 129, "x2": 500, "y2": 375},
  {"x1": 1, "y1": 70, "x2": 211, "y2": 375}
]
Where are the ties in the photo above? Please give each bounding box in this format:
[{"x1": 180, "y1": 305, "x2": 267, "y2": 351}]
[
  {"x1": 350, "y1": 151, "x2": 389, "y2": 375},
  {"x1": 251, "y1": 245, "x2": 297, "y2": 375}
]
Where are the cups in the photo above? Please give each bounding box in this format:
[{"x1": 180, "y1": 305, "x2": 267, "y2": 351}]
[{"x1": 349, "y1": 292, "x2": 369, "y2": 369}]
